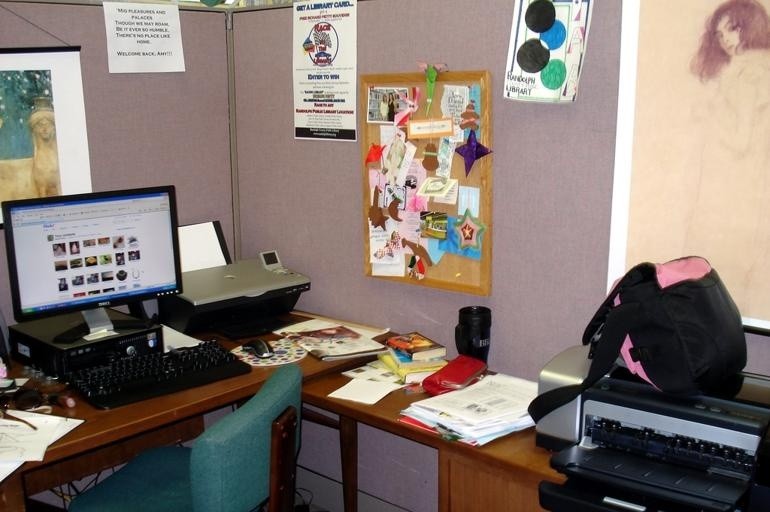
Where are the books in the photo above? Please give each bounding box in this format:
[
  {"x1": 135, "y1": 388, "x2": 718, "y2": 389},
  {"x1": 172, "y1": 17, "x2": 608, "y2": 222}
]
[{"x1": 279, "y1": 322, "x2": 449, "y2": 385}]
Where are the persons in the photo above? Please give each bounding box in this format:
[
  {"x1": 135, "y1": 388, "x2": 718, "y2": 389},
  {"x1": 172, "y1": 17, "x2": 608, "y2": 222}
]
[
  {"x1": 380, "y1": 94, "x2": 389, "y2": 121},
  {"x1": 686, "y1": 0, "x2": 770, "y2": 324},
  {"x1": 387, "y1": 92, "x2": 396, "y2": 121},
  {"x1": 28, "y1": 109, "x2": 60, "y2": 197}
]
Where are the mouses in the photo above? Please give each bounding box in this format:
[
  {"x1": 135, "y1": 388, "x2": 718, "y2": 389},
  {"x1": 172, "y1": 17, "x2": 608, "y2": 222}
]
[{"x1": 243, "y1": 338, "x2": 274, "y2": 358}]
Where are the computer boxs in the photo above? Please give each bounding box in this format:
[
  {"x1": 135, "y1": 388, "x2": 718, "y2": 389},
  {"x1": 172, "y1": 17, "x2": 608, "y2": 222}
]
[{"x1": 4, "y1": 309, "x2": 165, "y2": 378}]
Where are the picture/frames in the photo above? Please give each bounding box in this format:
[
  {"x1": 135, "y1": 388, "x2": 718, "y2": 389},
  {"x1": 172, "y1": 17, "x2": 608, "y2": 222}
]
[{"x1": 359, "y1": 70, "x2": 493, "y2": 297}]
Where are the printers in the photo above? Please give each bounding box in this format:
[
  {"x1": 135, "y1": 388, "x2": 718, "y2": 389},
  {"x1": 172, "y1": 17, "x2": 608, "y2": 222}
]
[
  {"x1": 156, "y1": 221, "x2": 312, "y2": 341},
  {"x1": 535, "y1": 315, "x2": 769, "y2": 511}
]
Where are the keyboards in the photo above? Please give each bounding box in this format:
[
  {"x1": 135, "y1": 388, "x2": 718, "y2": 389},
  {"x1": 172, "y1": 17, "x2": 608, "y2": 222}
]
[{"x1": 61, "y1": 339, "x2": 252, "y2": 410}]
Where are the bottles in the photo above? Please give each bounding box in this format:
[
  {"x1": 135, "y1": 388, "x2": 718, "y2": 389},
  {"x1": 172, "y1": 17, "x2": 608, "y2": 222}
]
[
  {"x1": 28, "y1": 96, "x2": 60, "y2": 197},
  {"x1": 72, "y1": 242, "x2": 77, "y2": 253},
  {"x1": 117, "y1": 270, "x2": 127, "y2": 281}
]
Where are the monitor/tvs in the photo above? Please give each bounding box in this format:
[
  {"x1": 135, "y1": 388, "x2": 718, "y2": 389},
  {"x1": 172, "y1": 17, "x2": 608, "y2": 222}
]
[{"x1": 1, "y1": 184, "x2": 184, "y2": 345}]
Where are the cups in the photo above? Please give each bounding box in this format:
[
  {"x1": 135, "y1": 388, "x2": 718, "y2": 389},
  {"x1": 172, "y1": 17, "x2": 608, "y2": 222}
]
[{"x1": 453, "y1": 305, "x2": 492, "y2": 366}]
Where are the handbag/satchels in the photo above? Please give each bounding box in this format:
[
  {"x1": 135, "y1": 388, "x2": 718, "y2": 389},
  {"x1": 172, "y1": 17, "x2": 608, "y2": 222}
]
[{"x1": 422, "y1": 354, "x2": 488, "y2": 396}]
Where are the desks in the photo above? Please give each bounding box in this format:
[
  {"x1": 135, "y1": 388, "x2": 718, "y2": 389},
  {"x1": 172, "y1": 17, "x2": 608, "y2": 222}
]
[
  {"x1": 0, "y1": 305, "x2": 399, "y2": 511},
  {"x1": 300, "y1": 358, "x2": 568, "y2": 511}
]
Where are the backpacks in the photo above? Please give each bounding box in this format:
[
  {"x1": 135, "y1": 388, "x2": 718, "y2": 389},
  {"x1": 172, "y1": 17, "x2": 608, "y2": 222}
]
[{"x1": 527, "y1": 255, "x2": 745, "y2": 424}]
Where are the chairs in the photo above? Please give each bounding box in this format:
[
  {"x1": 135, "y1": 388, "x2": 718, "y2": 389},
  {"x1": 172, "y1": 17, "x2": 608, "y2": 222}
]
[{"x1": 63, "y1": 362, "x2": 304, "y2": 512}]
